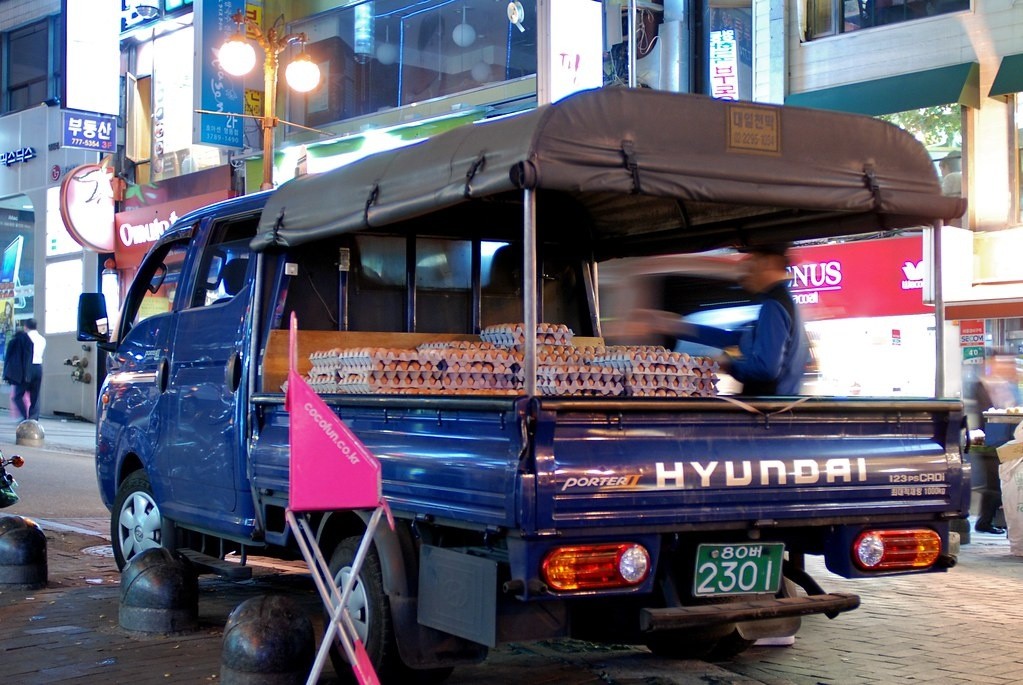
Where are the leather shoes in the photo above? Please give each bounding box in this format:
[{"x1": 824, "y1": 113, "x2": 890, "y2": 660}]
[{"x1": 974, "y1": 525, "x2": 1005, "y2": 534}]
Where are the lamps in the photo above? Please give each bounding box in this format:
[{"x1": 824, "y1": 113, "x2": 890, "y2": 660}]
[
  {"x1": 472, "y1": 35, "x2": 492, "y2": 81},
  {"x1": 44, "y1": 96, "x2": 61, "y2": 108},
  {"x1": 453, "y1": 3, "x2": 476, "y2": 48},
  {"x1": 377, "y1": 16, "x2": 398, "y2": 65}
]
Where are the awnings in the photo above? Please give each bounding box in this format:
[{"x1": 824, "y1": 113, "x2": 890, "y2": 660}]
[
  {"x1": 988, "y1": 53, "x2": 1023, "y2": 103},
  {"x1": 785, "y1": 62, "x2": 980, "y2": 116}
]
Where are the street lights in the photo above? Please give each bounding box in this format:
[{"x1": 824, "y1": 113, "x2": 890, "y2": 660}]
[{"x1": 216, "y1": 8, "x2": 322, "y2": 191}]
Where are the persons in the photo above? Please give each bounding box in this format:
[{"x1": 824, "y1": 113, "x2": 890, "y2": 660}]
[
  {"x1": 602, "y1": 232, "x2": 807, "y2": 396},
  {"x1": 12, "y1": 317, "x2": 46, "y2": 422},
  {"x1": 973, "y1": 350, "x2": 1017, "y2": 535}
]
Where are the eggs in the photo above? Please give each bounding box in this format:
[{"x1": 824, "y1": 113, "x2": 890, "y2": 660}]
[{"x1": 300, "y1": 322, "x2": 715, "y2": 396}]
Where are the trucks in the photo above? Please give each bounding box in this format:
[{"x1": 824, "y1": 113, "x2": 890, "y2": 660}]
[{"x1": 76, "y1": 84, "x2": 973, "y2": 685}]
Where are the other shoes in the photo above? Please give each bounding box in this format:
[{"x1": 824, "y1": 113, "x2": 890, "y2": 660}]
[{"x1": 19, "y1": 419, "x2": 26, "y2": 422}]
